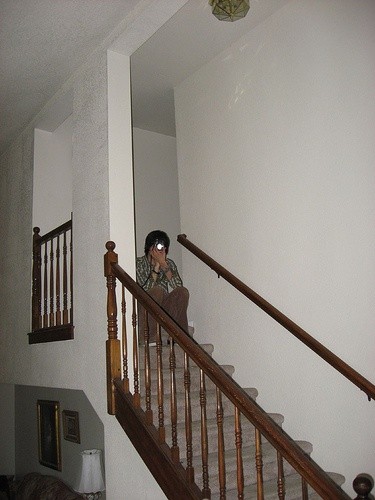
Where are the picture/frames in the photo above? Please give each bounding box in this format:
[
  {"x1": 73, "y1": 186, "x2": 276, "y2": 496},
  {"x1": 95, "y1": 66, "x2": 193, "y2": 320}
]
[
  {"x1": 62, "y1": 409, "x2": 80, "y2": 444},
  {"x1": 37, "y1": 400, "x2": 64, "y2": 473}
]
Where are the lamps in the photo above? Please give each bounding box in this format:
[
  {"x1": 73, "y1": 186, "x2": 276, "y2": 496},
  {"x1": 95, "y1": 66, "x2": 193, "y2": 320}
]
[
  {"x1": 75, "y1": 449, "x2": 106, "y2": 500},
  {"x1": 208, "y1": 0, "x2": 250, "y2": 22}
]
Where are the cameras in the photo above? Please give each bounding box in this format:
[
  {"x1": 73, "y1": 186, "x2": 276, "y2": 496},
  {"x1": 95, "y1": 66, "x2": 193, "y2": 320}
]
[{"x1": 155, "y1": 238, "x2": 164, "y2": 252}]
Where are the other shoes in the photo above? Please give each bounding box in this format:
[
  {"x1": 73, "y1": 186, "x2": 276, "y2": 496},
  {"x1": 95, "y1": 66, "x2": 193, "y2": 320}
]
[
  {"x1": 140, "y1": 327, "x2": 157, "y2": 348},
  {"x1": 167, "y1": 335, "x2": 175, "y2": 345}
]
